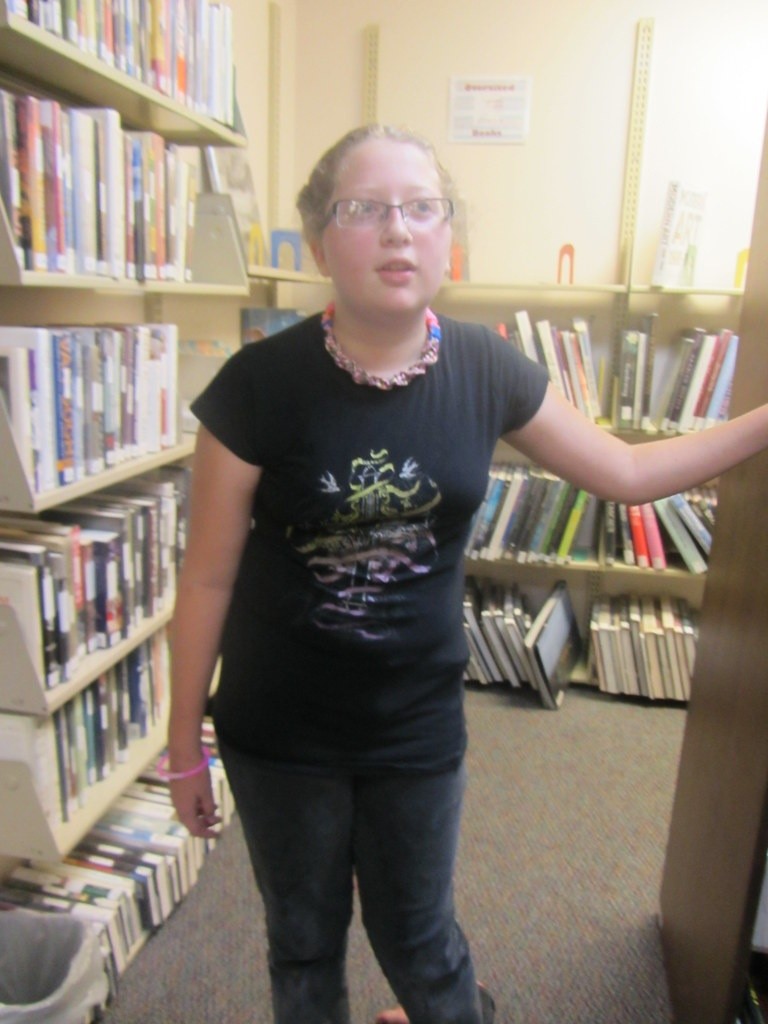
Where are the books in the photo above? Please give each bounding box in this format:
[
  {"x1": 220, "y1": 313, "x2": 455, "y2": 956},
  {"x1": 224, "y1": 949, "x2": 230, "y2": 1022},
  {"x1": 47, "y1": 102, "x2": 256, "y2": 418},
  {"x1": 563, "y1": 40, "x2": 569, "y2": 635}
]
[
  {"x1": 620, "y1": 314, "x2": 738, "y2": 434},
  {"x1": 1, "y1": 322, "x2": 178, "y2": 491},
  {"x1": 461, "y1": 459, "x2": 598, "y2": 563},
  {"x1": 0, "y1": 713, "x2": 237, "y2": 995},
  {"x1": 0, "y1": 469, "x2": 193, "y2": 687},
  {"x1": 462, "y1": 575, "x2": 582, "y2": 709},
  {"x1": 603, "y1": 485, "x2": 716, "y2": 575},
  {"x1": 589, "y1": 595, "x2": 701, "y2": 703},
  {"x1": 7, "y1": 0, "x2": 236, "y2": 127},
  {"x1": 239, "y1": 308, "x2": 304, "y2": 349},
  {"x1": 1, "y1": 627, "x2": 171, "y2": 823},
  {"x1": 1, "y1": 91, "x2": 203, "y2": 278},
  {"x1": 494, "y1": 310, "x2": 617, "y2": 424}
]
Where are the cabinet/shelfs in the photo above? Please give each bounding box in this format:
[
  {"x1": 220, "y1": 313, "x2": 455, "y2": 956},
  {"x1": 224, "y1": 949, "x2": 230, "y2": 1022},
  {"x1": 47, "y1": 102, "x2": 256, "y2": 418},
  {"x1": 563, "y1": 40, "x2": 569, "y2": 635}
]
[{"x1": 0, "y1": 0, "x2": 749, "y2": 968}]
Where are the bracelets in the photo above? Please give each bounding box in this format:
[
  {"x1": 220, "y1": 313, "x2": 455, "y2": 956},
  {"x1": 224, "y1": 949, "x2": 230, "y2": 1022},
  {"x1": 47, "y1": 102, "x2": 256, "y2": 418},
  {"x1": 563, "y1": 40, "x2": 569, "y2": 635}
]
[{"x1": 156, "y1": 746, "x2": 211, "y2": 779}]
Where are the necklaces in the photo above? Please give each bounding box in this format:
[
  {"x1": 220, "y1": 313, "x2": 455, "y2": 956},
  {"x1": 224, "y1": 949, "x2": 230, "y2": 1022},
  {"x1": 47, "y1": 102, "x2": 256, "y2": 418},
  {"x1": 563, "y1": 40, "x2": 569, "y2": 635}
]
[{"x1": 321, "y1": 301, "x2": 442, "y2": 390}]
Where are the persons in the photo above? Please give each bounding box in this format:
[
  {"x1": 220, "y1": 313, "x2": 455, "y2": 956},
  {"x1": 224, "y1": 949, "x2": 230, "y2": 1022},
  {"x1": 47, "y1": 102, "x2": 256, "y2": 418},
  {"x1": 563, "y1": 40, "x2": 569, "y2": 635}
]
[{"x1": 156, "y1": 123, "x2": 768, "y2": 1023}]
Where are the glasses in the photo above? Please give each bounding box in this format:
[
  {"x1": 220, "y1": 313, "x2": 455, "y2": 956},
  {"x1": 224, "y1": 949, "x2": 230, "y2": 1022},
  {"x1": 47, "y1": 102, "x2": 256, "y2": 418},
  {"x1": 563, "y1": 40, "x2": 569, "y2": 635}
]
[{"x1": 317, "y1": 197, "x2": 454, "y2": 235}]
